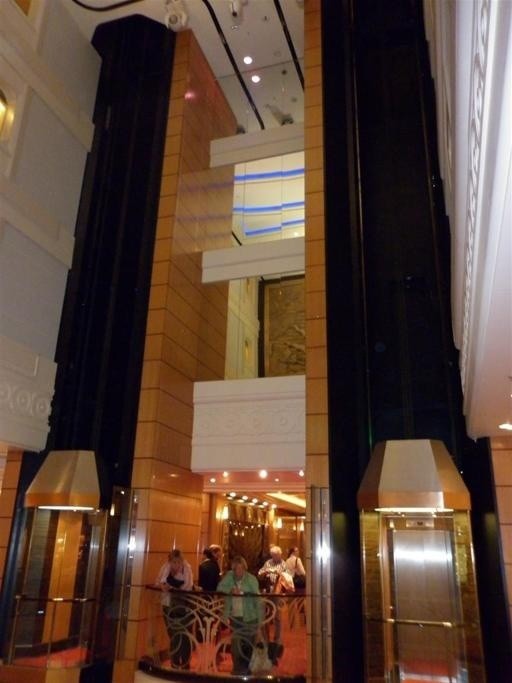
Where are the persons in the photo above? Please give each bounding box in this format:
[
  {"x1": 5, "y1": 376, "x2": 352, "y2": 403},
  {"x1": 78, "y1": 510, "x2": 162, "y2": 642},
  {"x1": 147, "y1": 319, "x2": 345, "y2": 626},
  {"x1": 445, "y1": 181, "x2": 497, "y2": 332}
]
[
  {"x1": 286, "y1": 546, "x2": 306, "y2": 629},
  {"x1": 197, "y1": 544, "x2": 222, "y2": 644},
  {"x1": 257, "y1": 546, "x2": 289, "y2": 643},
  {"x1": 154, "y1": 550, "x2": 195, "y2": 669},
  {"x1": 215, "y1": 555, "x2": 266, "y2": 675}
]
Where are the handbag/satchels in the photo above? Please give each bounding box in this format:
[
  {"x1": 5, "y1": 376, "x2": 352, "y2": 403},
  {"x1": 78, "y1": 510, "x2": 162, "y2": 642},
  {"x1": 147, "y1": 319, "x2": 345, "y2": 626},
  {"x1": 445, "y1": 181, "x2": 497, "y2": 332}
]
[
  {"x1": 248, "y1": 639, "x2": 272, "y2": 673},
  {"x1": 293, "y1": 571, "x2": 306, "y2": 588}
]
